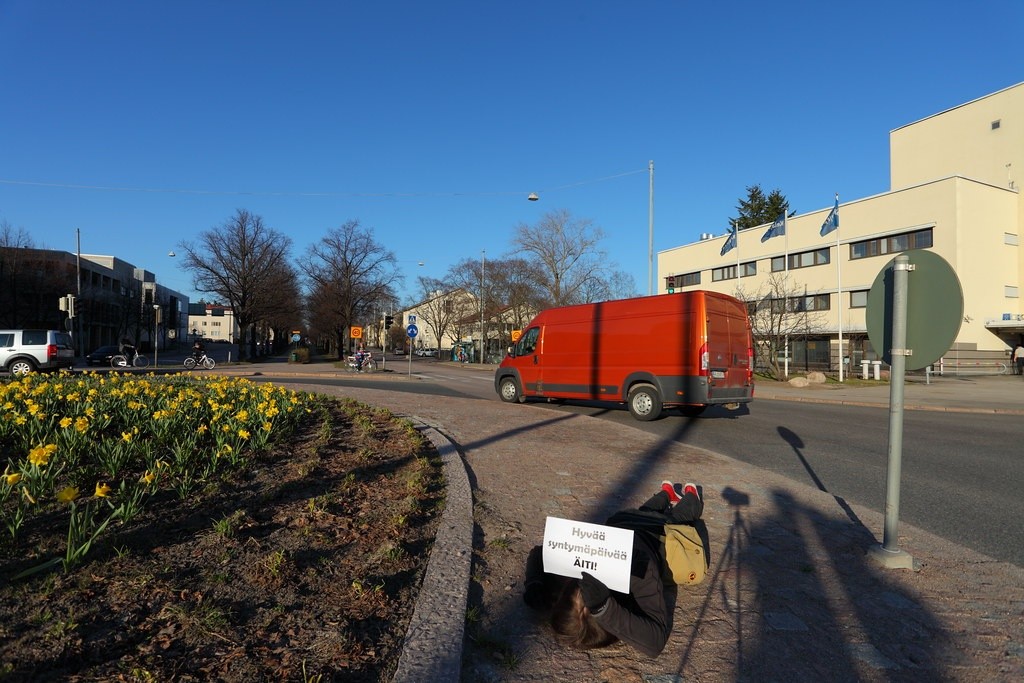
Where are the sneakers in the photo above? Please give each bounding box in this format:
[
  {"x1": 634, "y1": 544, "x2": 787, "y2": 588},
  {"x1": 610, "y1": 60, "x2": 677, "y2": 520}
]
[{"x1": 662, "y1": 480, "x2": 700, "y2": 507}]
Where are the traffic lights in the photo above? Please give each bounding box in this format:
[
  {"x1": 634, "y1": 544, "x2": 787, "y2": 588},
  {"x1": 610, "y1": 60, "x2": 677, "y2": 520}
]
[
  {"x1": 668, "y1": 276, "x2": 675, "y2": 294},
  {"x1": 385, "y1": 316, "x2": 394, "y2": 329}
]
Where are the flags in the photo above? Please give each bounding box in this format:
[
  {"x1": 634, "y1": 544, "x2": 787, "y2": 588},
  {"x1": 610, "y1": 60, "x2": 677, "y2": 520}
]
[
  {"x1": 761, "y1": 213, "x2": 784, "y2": 243},
  {"x1": 721, "y1": 226, "x2": 736, "y2": 256},
  {"x1": 820, "y1": 197, "x2": 839, "y2": 237}
]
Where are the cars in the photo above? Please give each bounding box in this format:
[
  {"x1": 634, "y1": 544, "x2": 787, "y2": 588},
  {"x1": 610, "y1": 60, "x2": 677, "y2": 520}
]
[
  {"x1": 86, "y1": 345, "x2": 122, "y2": 366},
  {"x1": 392, "y1": 347, "x2": 404, "y2": 355}
]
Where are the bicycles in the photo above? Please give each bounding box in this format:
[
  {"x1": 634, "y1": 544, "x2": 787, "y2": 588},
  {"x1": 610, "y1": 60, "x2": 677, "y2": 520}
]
[
  {"x1": 111, "y1": 346, "x2": 151, "y2": 369},
  {"x1": 184, "y1": 351, "x2": 216, "y2": 370},
  {"x1": 343, "y1": 353, "x2": 378, "y2": 373}
]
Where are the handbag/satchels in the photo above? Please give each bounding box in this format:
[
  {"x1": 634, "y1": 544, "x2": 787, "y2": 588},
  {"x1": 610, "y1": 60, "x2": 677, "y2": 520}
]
[{"x1": 660, "y1": 522, "x2": 708, "y2": 585}]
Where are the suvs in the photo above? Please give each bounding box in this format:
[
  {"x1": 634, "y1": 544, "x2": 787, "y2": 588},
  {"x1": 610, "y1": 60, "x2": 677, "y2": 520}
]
[
  {"x1": 418, "y1": 348, "x2": 438, "y2": 357},
  {"x1": 0, "y1": 329, "x2": 75, "y2": 377}
]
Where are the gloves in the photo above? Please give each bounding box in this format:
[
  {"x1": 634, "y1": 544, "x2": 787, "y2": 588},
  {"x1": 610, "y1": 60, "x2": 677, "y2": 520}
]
[{"x1": 581, "y1": 572, "x2": 609, "y2": 613}]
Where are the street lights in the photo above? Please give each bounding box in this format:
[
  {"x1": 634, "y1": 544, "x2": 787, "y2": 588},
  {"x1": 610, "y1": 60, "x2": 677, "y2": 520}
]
[{"x1": 153, "y1": 305, "x2": 159, "y2": 368}]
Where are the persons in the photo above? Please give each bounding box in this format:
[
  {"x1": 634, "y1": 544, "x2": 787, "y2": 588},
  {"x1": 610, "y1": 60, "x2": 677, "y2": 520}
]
[
  {"x1": 120, "y1": 334, "x2": 136, "y2": 368},
  {"x1": 457, "y1": 346, "x2": 465, "y2": 364},
  {"x1": 357, "y1": 344, "x2": 366, "y2": 374},
  {"x1": 523, "y1": 480, "x2": 704, "y2": 658},
  {"x1": 192, "y1": 341, "x2": 201, "y2": 366}
]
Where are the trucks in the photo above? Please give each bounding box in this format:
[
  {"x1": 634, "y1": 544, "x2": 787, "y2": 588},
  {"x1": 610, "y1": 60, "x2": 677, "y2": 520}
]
[{"x1": 495, "y1": 290, "x2": 754, "y2": 422}]
